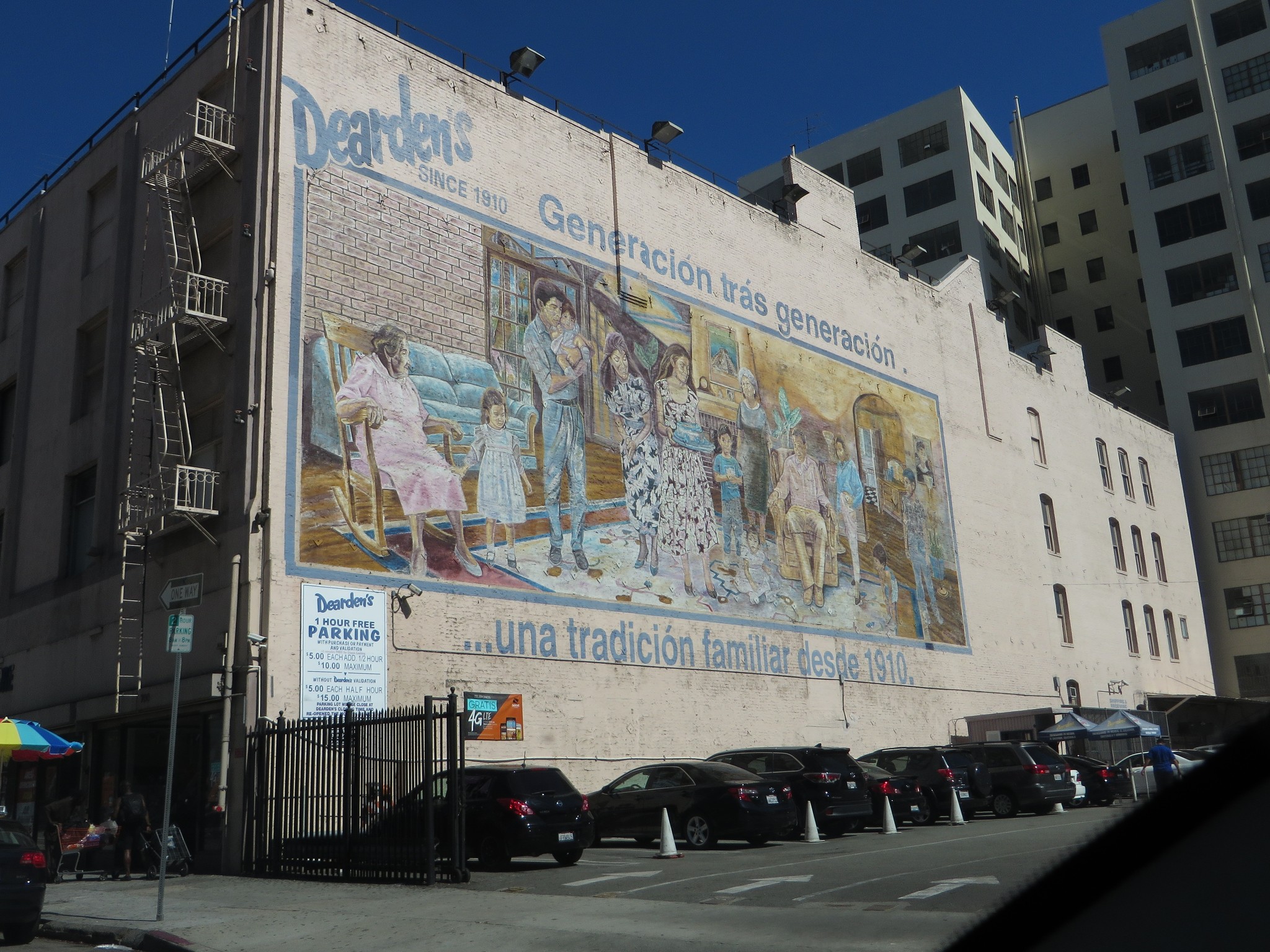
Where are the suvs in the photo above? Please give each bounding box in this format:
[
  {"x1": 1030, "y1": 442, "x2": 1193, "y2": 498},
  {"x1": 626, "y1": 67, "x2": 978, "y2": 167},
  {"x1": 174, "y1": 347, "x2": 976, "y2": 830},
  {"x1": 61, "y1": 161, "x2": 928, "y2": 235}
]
[
  {"x1": 942, "y1": 738, "x2": 1077, "y2": 819},
  {"x1": 854, "y1": 746, "x2": 994, "y2": 827},
  {"x1": 703, "y1": 742, "x2": 870, "y2": 841}
]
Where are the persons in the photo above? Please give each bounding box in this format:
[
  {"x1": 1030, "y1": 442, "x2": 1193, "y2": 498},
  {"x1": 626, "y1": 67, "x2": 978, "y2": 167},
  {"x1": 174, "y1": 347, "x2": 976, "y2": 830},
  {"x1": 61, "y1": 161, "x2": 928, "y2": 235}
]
[
  {"x1": 35, "y1": 799, "x2": 62, "y2": 883},
  {"x1": 111, "y1": 780, "x2": 155, "y2": 881},
  {"x1": 1140, "y1": 738, "x2": 1182, "y2": 792}
]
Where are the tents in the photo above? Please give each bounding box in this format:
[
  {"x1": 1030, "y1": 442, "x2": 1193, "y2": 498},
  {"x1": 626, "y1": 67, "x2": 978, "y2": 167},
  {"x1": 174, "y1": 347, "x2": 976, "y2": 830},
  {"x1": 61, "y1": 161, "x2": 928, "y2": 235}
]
[
  {"x1": 1038, "y1": 711, "x2": 1099, "y2": 755},
  {"x1": 1086, "y1": 709, "x2": 1164, "y2": 799}
]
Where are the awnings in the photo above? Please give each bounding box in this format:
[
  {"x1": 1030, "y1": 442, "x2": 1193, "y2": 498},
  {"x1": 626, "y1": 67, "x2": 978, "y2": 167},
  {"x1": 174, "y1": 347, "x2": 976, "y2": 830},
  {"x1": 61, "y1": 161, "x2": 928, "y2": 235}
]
[{"x1": 1144, "y1": 692, "x2": 1270, "y2": 714}]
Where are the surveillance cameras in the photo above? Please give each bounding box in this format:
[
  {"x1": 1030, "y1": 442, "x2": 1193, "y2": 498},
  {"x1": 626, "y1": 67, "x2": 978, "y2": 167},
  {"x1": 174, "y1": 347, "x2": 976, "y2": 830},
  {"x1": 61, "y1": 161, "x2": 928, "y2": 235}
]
[{"x1": 246, "y1": 633, "x2": 267, "y2": 649}]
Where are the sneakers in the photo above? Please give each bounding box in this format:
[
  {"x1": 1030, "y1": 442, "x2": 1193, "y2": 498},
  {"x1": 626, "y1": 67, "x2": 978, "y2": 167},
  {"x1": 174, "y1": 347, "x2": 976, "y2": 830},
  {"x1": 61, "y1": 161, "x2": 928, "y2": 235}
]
[{"x1": 121, "y1": 875, "x2": 132, "y2": 881}]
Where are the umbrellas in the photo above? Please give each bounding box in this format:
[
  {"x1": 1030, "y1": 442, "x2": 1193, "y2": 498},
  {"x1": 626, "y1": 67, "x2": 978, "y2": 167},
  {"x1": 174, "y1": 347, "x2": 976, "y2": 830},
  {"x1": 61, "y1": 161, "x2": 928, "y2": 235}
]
[{"x1": 0, "y1": 716, "x2": 86, "y2": 779}]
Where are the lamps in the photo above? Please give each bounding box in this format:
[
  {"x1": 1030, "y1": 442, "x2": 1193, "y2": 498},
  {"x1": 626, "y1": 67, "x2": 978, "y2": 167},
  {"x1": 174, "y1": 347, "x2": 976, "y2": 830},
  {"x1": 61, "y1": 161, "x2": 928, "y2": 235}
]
[
  {"x1": 646, "y1": 121, "x2": 684, "y2": 145},
  {"x1": 774, "y1": 184, "x2": 809, "y2": 205},
  {"x1": 894, "y1": 244, "x2": 927, "y2": 260},
  {"x1": 1029, "y1": 345, "x2": 1057, "y2": 357},
  {"x1": 1107, "y1": 386, "x2": 1131, "y2": 398},
  {"x1": 506, "y1": 47, "x2": 546, "y2": 79},
  {"x1": 990, "y1": 289, "x2": 1020, "y2": 306}
]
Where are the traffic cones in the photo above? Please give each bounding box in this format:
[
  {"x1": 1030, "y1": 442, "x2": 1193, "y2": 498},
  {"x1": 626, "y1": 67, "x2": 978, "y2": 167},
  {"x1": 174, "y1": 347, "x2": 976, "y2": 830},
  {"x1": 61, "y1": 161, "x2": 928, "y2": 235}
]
[
  {"x1": 943, "y1": 788, "x2": 967, "y2": 826},
  {"x1": 799, "y1": 801, "x2": 825, "y2": 844},
  {"x1": 653, "y1": 808, "x2": 685, "y2": 860},
  {"x1": 876, "y1": 796, "x2": 902, "y2": 835}
]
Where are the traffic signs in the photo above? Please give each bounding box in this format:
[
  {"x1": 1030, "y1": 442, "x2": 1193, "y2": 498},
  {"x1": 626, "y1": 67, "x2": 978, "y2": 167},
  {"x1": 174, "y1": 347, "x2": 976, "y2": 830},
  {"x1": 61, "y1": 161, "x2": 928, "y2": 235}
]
[{"x1": 159, "y1": 573, "x2": 204, "y2": 653}]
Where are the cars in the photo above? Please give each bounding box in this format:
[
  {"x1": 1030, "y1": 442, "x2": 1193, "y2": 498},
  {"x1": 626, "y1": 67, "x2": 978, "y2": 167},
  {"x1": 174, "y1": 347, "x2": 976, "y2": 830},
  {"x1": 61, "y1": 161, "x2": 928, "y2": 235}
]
[
  {"x1": 368, "y1": 764, "x2": 592, "y2": 871},
  {"x1": 1056, "y1": 754, "x2": 1133, "y2": 808},
  {"x1": 1069, "y1": 769, "x2": 1086, "y2": 799},
  {"x1": 0, "y1": 817, "x2": 46, "y2": 942},
  {"x1": 1112, "y1": 749, "x2": 1209, "y2": 798},
  {"x1": 844, "y1": 760, "x2": 923, "y2": 833},
  {"x1": 579, "y1": 761, "x2": 797, "y2": 851}
]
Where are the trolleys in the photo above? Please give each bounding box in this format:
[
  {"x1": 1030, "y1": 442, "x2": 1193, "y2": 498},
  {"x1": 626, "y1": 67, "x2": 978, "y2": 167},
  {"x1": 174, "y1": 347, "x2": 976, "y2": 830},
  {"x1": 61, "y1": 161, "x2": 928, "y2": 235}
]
[{"x1": 52, "y1": 823, "x2": 191, "y2": 883}]
